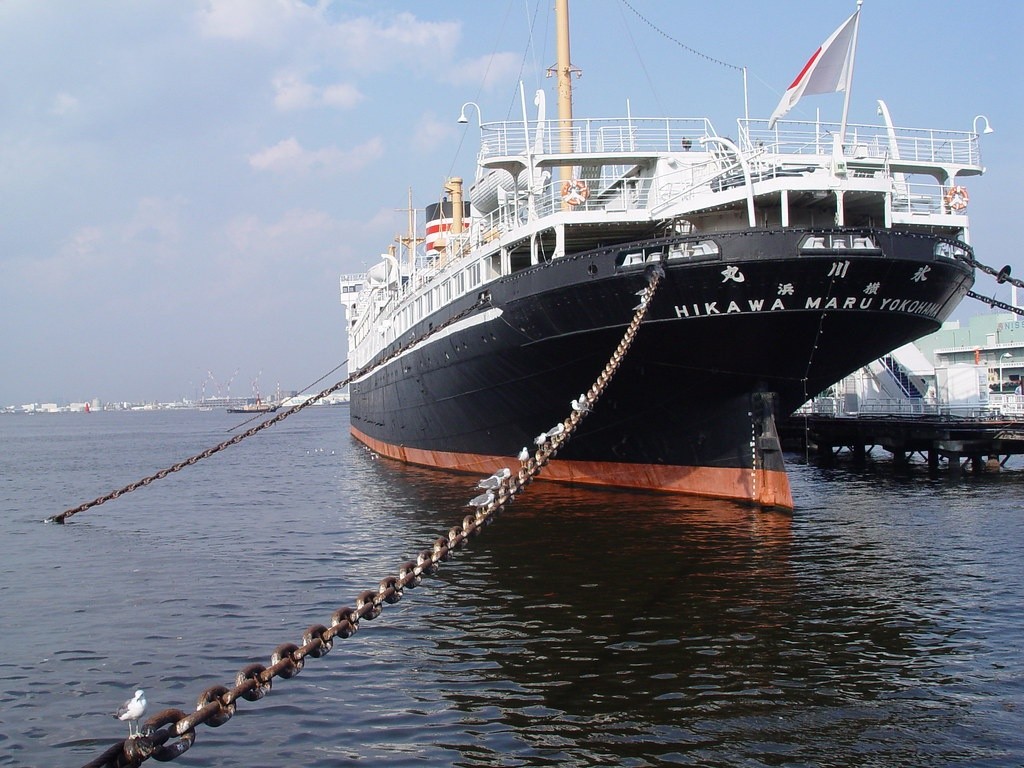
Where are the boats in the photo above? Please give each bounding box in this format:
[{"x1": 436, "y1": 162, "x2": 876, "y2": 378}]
[{"x1": 227, "y1": 371, "x2": 349, "y2": 415}]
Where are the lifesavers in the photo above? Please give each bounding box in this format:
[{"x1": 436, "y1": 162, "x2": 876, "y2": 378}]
[
  {"x1": 562, "y1": 180, "x2": 589, "y2": 206},
  {"x1": 947, "y1": 187, "x2": 969, "y2": 210}
]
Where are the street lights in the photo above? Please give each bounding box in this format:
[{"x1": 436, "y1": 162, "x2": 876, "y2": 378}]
[
  {"x1": 1000, "y1": 353, "x2": 1014, "y2": 393},
  {"x1": 456, "y1": 102, "x2": 484, "y2": 144},
  {"x1": 973, "y1": 115, "x2": 993, "y2": 165}
]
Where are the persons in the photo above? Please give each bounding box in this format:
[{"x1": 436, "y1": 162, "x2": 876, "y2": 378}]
[
  {"x1": 984, "y1": 454, "x2": 1000, "y2": 474},
  {"x1": 1015, "y1": 380, "x2": 1022, "y2": 396}
]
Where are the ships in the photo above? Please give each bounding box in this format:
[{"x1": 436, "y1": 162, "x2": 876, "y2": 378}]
[{"x1": 340, "y1": 0, "x2": 994, "y2": 511}]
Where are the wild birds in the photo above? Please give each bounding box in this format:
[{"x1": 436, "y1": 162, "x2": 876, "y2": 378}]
[
  {"x1": 110, "y1": 689, "x2": 147, "y2": 740},
  {"x1": 632, "y1": 287, "x2": 649, "y2": 313},
  {"x1": 466, "y1": 393, "x2": 593, "y2": 511}
]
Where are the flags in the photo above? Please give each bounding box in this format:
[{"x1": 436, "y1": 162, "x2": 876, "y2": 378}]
[{"x1": 768, "y1": 8, "x2": 860, "y2": 130}]
